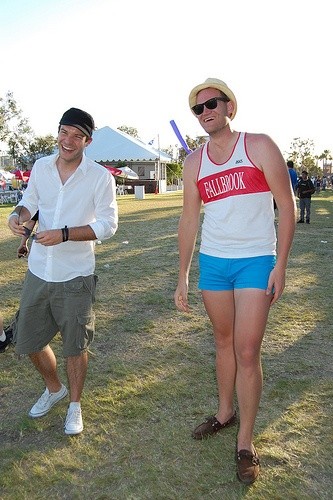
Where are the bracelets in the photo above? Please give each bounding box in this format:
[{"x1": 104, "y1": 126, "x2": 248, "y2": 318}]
[
  {"x1": 9, "y1": 212, "x2": 21, "y2": 220},
  {"x1": 62, "y1": 226, "x2": 69, "y2": 242}
]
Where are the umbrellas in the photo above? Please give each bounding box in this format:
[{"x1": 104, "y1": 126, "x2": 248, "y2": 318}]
[{"x1": 115, "y1": 166, "x2": 139, "y2": 179}]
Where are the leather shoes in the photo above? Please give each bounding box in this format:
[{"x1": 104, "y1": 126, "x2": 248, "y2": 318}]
[
  {"x1": 235, "y1": 440, "x2": 260, "y2": 484},
  {"x1": 191, "y1": 410, "x2": 237, "y2": 440}
]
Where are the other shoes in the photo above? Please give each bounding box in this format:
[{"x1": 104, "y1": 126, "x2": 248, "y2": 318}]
[
  {"x1": 297, "y1": 218, "x2": 304, "y2": 223},
  {"x1": 306, "y1": 220, "x2": 310, "y2": 224}
]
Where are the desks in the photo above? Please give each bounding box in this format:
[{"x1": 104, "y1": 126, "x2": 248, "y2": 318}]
[
  {"x1": 116, "y1": 186, "x2": 132, "y2": 195},
  {"x1": 134, "y1": 186, "x2": 145, "y2": 199}
]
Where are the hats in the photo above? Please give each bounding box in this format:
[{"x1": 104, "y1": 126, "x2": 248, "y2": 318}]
[
  {"x1": 189, "y1": 78, "x2": 237, "y2": 121},
  {"x1": 60, "y1": 107, "x2": 94, "y2": 139}
]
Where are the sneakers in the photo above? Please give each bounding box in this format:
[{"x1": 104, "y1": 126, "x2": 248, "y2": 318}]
[
  {"x1": 28, "y1": 383, "x2": 68, "y2": 418},
  {"x1": 0, "y1": 325, "x2": 13, "y2": 354},
  {"x1": 64, "y1": 402, "x2": 83, "y2": 434}
]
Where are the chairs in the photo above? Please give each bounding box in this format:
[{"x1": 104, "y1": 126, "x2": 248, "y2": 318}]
[{"x1": 117, "y1": 184, "x2": 124, "y2": 196}]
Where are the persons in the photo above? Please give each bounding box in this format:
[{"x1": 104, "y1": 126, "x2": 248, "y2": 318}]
[
  {"x1": 174, "y1": 78, "x2": 296, "y2": 483},
  {"x1": 0, "y1": 108, "x2": 119, "y2": 433},
  {"x1": 286, "y1": 159, "x2": 333, "y2": 224}
]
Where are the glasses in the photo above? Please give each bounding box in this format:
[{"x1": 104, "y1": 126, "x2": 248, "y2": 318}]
[
  {"x1": 191, "y1": 97, "x2": 230, "y2": 115},
  {"x1": 18, "y1": 205, "x2": 39, "y2": 240}
]
